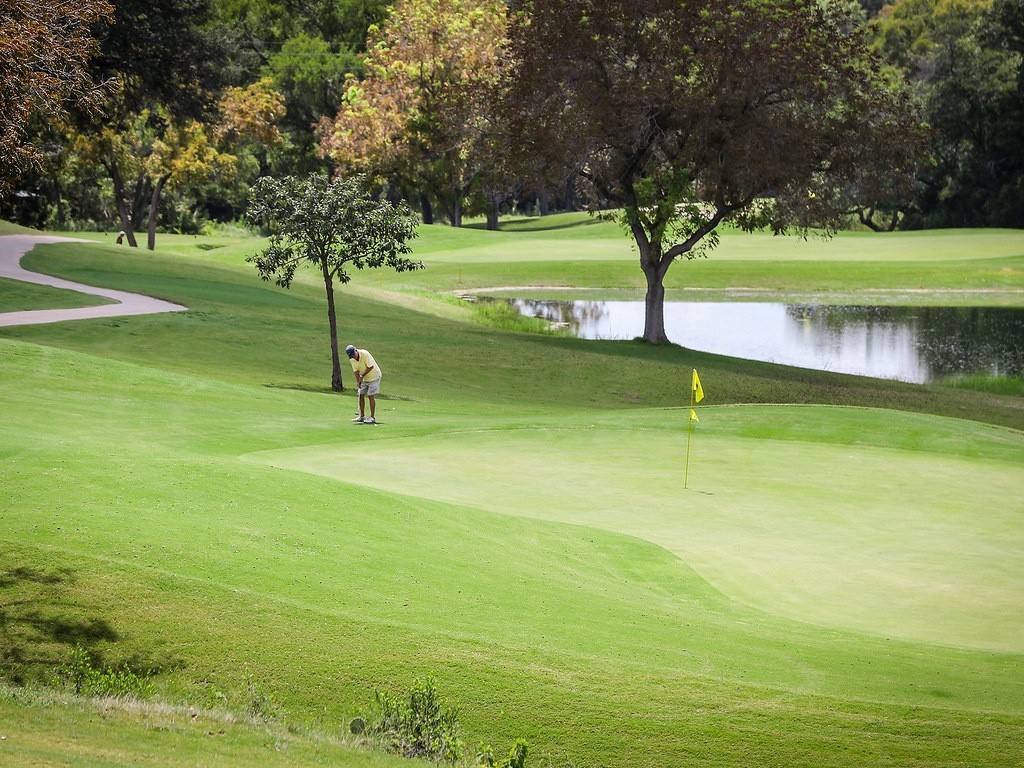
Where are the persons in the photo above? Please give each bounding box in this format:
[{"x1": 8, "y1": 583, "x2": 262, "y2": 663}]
[
  {"x1": 346, "y1": 345, "x2": 383, "y2": 424},
  {"x1": 116, "y1": 231, "x2": 126, "y2": 244}
]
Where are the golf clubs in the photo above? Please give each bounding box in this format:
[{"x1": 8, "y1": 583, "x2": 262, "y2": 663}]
[{"x1": 355, "y1": 381, "x2": 360, "y2": 415}]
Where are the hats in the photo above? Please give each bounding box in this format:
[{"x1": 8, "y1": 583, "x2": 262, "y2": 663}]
[{"x1": 346, "y1": 345, "x2": 356, "y2": 359}]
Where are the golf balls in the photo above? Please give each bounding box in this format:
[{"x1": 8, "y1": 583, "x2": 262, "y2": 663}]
[
  {"x1": 393, "y1": 408, "x2": 395, "y2": 410},
  {"x1": 375, "y1": 424, "x2": 377, "y2": 427}
]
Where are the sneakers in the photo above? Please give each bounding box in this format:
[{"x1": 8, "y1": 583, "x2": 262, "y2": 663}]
[{"x1": 351, "y1": 415, "x2": 375, "y2": 423}]
